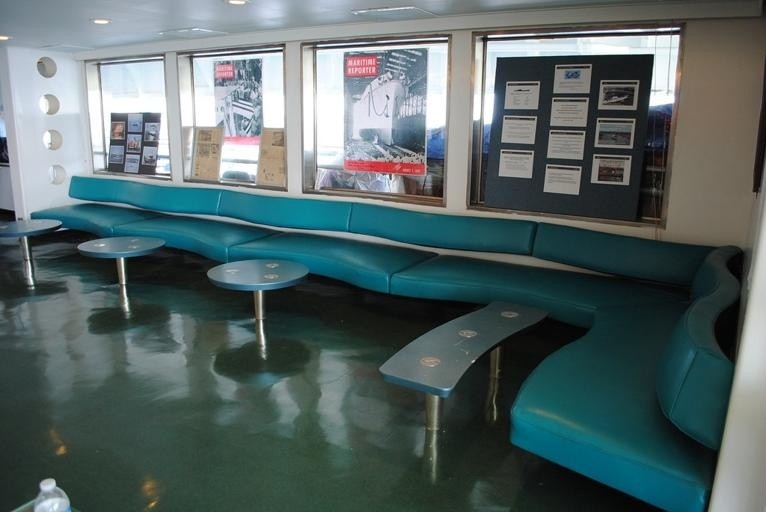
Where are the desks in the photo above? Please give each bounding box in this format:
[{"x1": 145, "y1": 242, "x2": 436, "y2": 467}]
[{"x1": 0, "y1": 218, "x2": 63, "y2": 292}]
